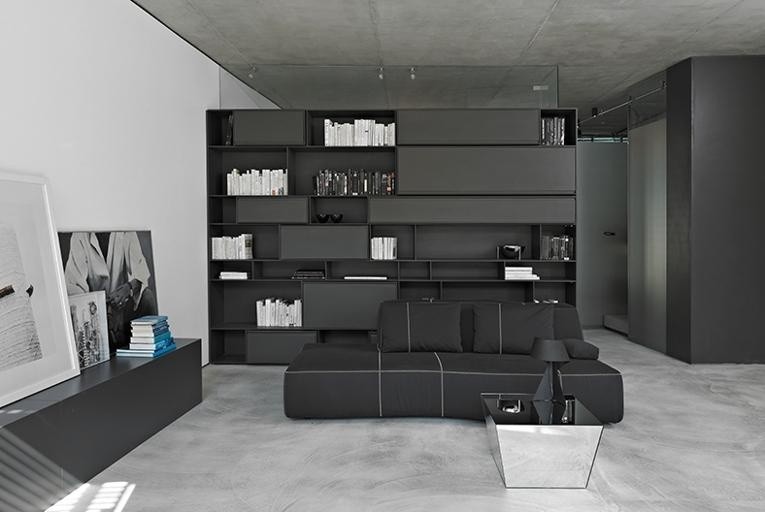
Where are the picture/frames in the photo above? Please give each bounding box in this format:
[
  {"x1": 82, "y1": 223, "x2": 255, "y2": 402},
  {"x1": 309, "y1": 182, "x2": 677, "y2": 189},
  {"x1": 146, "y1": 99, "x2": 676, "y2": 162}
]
[{"x1": 0, "y1": 172, "x2": 82, "y2": 408}]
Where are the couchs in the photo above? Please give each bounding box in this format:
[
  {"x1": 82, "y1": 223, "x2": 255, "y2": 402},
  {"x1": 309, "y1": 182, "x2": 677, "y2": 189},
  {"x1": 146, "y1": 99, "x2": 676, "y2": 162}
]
[{"x1": 284, "y1": 297, "x2": 623, "y2": 423}]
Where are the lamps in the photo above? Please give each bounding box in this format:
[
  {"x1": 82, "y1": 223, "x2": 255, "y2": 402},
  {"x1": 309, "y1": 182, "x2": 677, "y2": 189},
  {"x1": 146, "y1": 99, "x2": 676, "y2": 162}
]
[{"x1": 531, "y1": 338, "x2": 571, "y2": 407}]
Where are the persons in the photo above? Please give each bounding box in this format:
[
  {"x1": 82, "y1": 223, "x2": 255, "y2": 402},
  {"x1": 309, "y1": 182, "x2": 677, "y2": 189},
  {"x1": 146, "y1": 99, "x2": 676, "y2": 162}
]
[{"x1": 63, "y1": 232, "x2": 157, "y2": 348}]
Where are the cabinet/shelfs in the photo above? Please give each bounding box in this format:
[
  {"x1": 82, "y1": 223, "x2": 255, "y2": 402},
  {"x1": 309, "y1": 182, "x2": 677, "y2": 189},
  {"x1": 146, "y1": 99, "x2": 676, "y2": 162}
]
[
  {"x1": 204, "y1": 107, "x2": 579, "y2": 366},
  {"x1": 0, "y1": 337, "x2": 202, "y2": 512}
]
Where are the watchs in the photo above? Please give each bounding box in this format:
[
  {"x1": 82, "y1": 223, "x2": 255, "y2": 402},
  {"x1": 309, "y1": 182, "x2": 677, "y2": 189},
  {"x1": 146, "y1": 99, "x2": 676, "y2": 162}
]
[{"x1": 126, "y1": 281, "x2": 134, "y2": 298}]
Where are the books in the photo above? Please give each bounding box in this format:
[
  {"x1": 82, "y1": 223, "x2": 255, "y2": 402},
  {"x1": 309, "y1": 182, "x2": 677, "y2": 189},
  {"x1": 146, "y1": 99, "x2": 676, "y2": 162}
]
[
  {"x1": 370, "y1": 236, "x2": 398, "y2": 261},
  {"x1": 541, "y1": 234, "x2": 574, "y2": 261},
  {"x1": 115, "y1": 315, "x2": 175, "y2": 357},
  {"x1": 505, "y1": 266, "x2": 542, "y2": 281},
  {"x1": 219, "y1": 276, "x2": 250, "y2": 280},
  {"x1": 315, "y1": 112, "x2": 399, "y2": 197},
  {"x1": 541, "y1": 117, "x2": 566, "y2": 147},
  {"x1": 210, "y1": 233, "x2": 254, "y2": 260},
  {"x1": 255, "y1": 297, "x2": 304, "y2": 328},
  {"x1": 291, "y1": 271, "x2": 325, "y2": 280},
  {"x1": 226, "y1": 166, "x2": 289, "y2": 198},
  {"x1": 219, "y1": 271, "x2": 249, "y2": 276},
  {"x1": 343, "y1": 275, "x2": 388, "y2": 281}
]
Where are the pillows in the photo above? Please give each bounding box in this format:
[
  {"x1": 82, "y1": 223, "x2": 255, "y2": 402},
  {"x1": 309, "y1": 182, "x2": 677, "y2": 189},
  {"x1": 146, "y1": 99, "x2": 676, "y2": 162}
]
[
  {"x1": 473, "y1": 302, "x2": 556, "y2": 353},
  {"x1": 379, "y1": 301, "x2": 463, "y2": 352}
]
